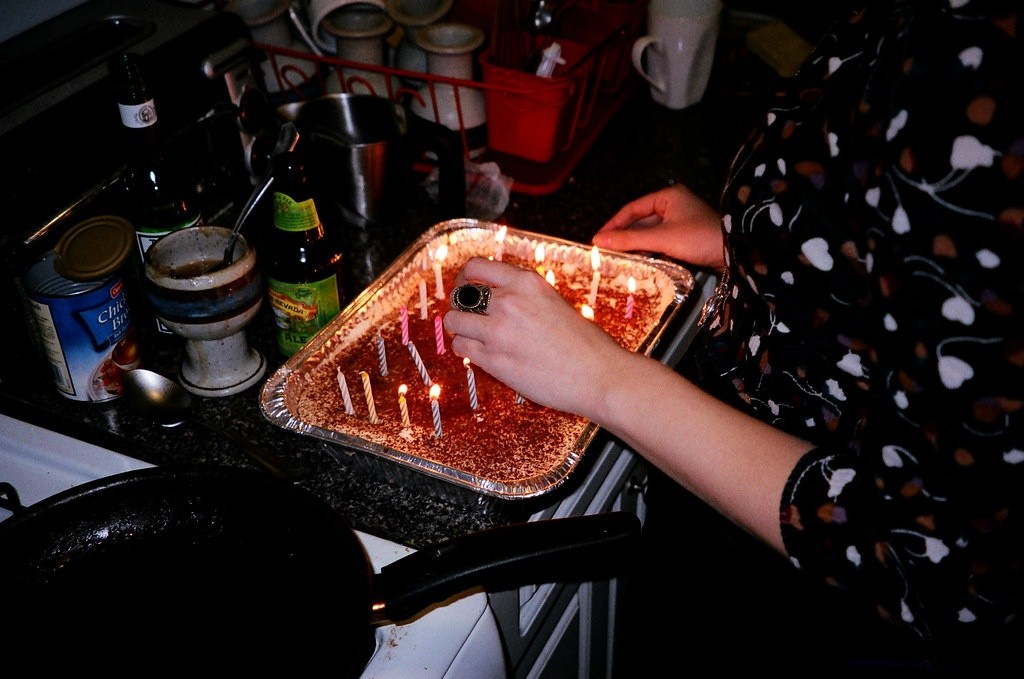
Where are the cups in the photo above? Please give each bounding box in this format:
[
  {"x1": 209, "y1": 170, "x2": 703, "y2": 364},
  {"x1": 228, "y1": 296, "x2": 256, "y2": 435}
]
[
  {"x1": 295, "y1": 93, "x2": 401, "y2": 226},
  {"x1": 631, "y1": 0, "x2": 722, "y2": 109},
  {"x1": 143, "y1": 225, "x2": 270, "y2": 398}
]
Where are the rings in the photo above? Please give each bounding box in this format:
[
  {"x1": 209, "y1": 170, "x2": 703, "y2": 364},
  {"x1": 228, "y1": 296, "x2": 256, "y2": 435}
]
[{"x1": 452, "y1": 285, "x2": 491, "y2": 315}]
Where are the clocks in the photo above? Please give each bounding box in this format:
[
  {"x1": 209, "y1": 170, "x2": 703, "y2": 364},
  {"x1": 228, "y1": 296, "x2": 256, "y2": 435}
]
[{"x1": 535, "y1": 245, "x2": 546, "y2": 278}]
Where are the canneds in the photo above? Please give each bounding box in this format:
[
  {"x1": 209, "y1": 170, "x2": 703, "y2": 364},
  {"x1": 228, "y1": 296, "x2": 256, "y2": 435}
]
[{"x1": 22, "y1": 248, "x2": 140, "y2": 406}]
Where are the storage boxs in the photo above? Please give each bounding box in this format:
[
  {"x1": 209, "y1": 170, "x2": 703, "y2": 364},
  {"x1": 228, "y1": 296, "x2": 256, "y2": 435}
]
[{"x1": 449, "y1": 0, "x2": 644, "y2": 163}]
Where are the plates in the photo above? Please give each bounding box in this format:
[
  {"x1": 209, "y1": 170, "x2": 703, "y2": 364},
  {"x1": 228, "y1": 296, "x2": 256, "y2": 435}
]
[{"x1": 258, "y1": 217, "x2": 695, "y2": 503}]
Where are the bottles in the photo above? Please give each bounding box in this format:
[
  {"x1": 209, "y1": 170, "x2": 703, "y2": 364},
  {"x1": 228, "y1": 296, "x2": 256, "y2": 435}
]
[
  {"x1": 221, "y1": 1, "x2": 490, "y2": 189},
  {"x1": 267, "y1": 161, "x2": 343, "y2": 361},
  {"x1": 111, "y1": 51, "x2": 162, "y2": 160},
  {"x1": 22, "y1": 248, "x2": 140, "y2": 404}
]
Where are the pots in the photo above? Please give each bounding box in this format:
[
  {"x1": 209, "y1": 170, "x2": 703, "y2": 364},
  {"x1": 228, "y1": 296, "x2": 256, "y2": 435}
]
[{"x1": 0, "y1": 464, "x2": 642, "y2": 679}]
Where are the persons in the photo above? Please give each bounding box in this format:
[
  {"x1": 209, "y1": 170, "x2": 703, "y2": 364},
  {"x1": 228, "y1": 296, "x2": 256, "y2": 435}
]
[{"x1": 442, "y1": 0, "x2": 1024, "y2": 679}]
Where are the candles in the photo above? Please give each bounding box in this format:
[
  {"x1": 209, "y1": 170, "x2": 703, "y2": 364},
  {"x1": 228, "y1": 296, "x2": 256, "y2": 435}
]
[
  {"x1": 376, "y1": 329, "x2": 388, "y2": 376},
  {"x1": 434, "y1": 243, "x2": 449, "y2": 300},
  {"x1": 359, "y1": 371, "x2": 379, "y2": 424},
  {"x1": 463, "y1": 357, "x2": 478, "y2": 409},
  {"x1": 336, "y1": 366, "x2": 356, "y2": 415},
  {"x1": 419, "y1": 278, "x2": 428, "y2": 320},
  {"x1": 400, "y1": 304, "x2": 409, "y2": 345},
  {"x1": 433, "y1": 310, "x2": 445, "y2": 355},
  {"x1": 429, "y1": 384, "x2": 443, "y2": 438},
  {"x1": 625, "y1": 276, "x2": 636, "y2": 317},
  {"x1": 589, "y1": 245, "x2": 601, "y2": 305},
  {"x1": 408, "y1": 341, "x2": 433, "y2": 385},
  {"x1": 398, "y1": 384, "x2": 411, "y2": 427},
  {"x1": 494, "y1": 225, "x2": 508, "y2": 262}
]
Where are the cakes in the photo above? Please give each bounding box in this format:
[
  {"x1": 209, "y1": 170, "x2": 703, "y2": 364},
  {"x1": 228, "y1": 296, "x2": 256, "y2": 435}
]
[{"x1": 295, "y1": 253, "x2": 661, "y2": 483}]
